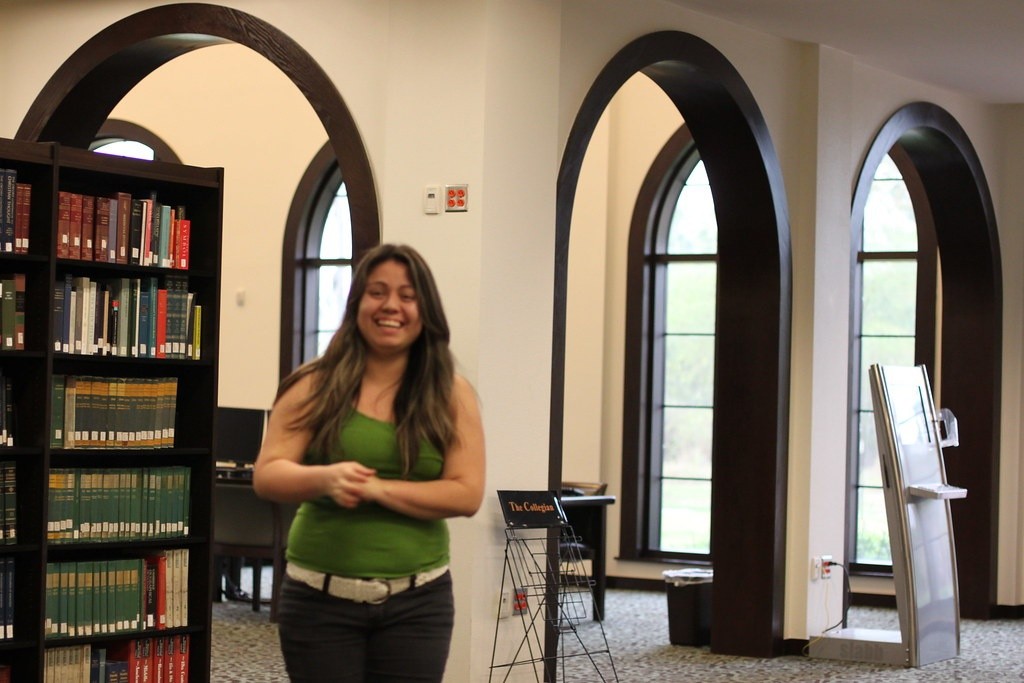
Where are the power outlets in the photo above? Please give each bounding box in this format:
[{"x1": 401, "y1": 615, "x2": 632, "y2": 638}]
[{"x1": 821, "y1": 556, "x2": 834, "y2": 579}]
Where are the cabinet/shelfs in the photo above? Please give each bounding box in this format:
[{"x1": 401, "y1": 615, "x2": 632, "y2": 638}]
[
  {"x1": 0, "y1": 136, "x2": 223, "y2": 683},
  {"x1": 486, "y1": 489, "x2": 620, "y2": 683}
]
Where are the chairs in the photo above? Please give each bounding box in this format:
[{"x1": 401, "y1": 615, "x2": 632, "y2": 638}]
[{"x1": 213, "y1": 407, "x2": 297, "y2": 619}]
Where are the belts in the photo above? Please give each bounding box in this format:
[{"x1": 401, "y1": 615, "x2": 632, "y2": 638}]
[{"x1": 284, "y1": 560, "x2": 449, "y2": 604}]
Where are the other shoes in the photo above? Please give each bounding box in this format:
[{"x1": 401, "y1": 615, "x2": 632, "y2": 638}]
[{"x1": 227, "y1": 589, "x2": 251, "y2": 602}]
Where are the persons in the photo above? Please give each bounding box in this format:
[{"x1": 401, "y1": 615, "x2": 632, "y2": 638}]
[{"x1": 251, "y1": 243, "x2": 487, "y2": 682}]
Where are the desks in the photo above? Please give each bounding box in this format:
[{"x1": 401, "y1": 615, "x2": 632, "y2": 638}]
[{"x1": 555, "y1": 496, "x2": 617, "y2": 622}]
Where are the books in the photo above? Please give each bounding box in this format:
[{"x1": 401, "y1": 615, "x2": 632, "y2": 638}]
[{"x1": 1, "y1": 164, "x2": 194, "y2": 683}]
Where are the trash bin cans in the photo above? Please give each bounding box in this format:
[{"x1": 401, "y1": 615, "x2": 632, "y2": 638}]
[{"x1": 661, "y1": 567, "x2": 714, "y2": 649}]
